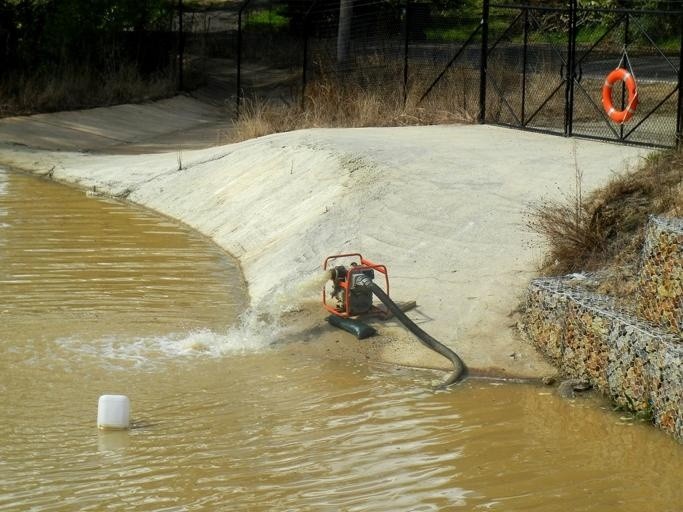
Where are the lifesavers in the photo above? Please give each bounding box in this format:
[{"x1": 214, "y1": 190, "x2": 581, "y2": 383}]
[{"x1": 602, "y1": 69, "x2": 637, "y2": 124}]
